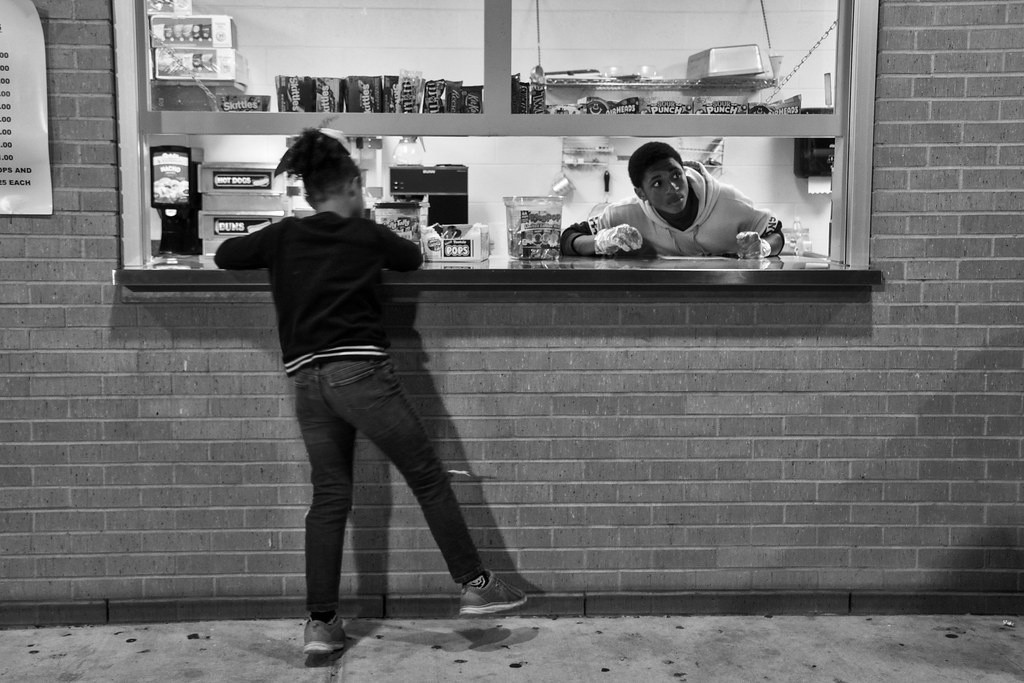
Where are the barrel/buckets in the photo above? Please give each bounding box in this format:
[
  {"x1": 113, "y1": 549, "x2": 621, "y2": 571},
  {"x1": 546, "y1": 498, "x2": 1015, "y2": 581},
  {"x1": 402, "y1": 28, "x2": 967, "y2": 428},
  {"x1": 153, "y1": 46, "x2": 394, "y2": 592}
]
[
  {"x1": 502, "y1": 196, "x2": 568, "y2": 261},
  {"x1": 373, "y1": 202, "x2": 422, "y2": 249}
]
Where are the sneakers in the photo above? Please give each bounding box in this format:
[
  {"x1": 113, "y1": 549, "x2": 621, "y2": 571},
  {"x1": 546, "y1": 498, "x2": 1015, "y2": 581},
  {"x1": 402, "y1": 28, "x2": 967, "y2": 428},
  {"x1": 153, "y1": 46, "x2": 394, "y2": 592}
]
[
  {"x1": 304, "y1": 617, "x2": 347, "y2": 655},
  {"x1": 458, "y1": 567, "x2": 527, "y2": 615}
]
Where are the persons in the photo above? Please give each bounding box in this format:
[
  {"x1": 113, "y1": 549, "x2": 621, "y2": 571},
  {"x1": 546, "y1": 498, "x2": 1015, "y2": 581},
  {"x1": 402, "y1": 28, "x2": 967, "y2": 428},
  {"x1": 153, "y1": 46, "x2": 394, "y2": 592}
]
[
  {"x1": 560, "y1": 142, "x2": 786, "y2": 260},
  {"x1": 213, "y1": 131, "x2": 532, "y2": 655}
]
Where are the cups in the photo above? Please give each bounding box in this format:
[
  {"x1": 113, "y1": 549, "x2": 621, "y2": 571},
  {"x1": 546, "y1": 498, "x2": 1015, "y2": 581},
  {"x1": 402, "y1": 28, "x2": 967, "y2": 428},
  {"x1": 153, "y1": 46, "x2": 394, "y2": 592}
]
[{"x1": 552, "y1": 174, "x2": 576, "y2": 197}]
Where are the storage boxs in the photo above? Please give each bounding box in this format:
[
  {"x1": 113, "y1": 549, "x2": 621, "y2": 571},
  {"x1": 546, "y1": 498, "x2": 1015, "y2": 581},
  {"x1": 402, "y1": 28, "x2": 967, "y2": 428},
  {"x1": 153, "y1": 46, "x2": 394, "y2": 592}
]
[{"x1": 151, "y1": 14, "x2": 248, "y2": 85}]
